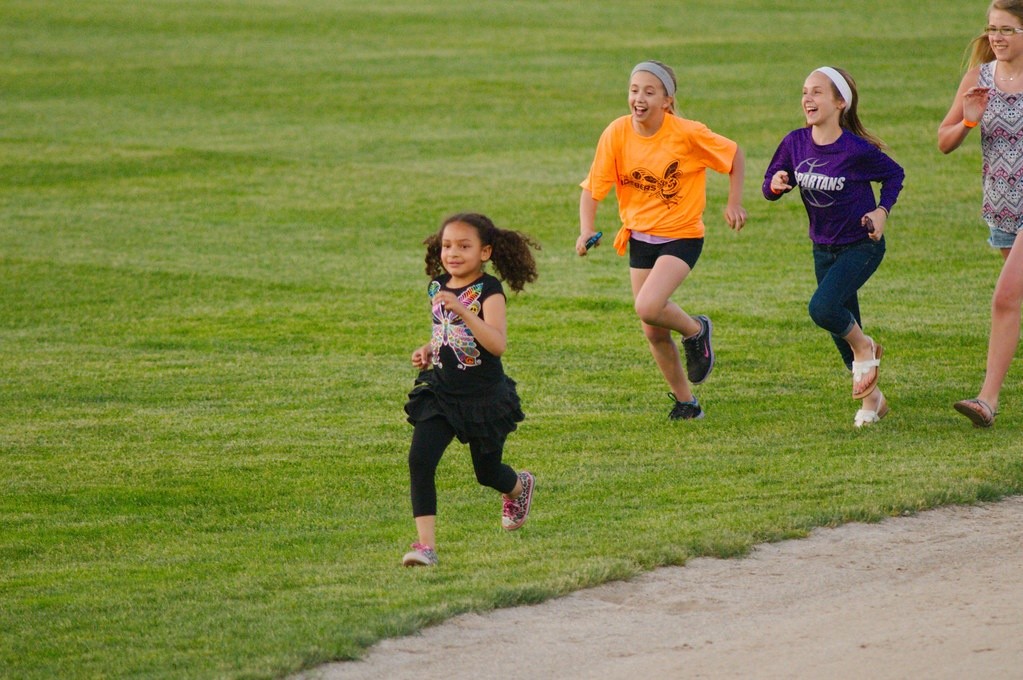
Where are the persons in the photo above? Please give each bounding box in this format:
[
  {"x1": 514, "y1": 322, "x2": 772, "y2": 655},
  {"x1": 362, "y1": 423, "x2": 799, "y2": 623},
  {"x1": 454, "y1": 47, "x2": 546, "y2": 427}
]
[
  {"x1": 402, "y1": 214, "x2": 542, "y2": 566},
  {"x1": 938, "y1": 0, "x2": 1023, "y2": 427},
  {"x1": 576, "y1": 60, "x2": 747, "y2": 421},
  {"x1": 762, "y1": 66, "x2": 905, "y2": 428}
]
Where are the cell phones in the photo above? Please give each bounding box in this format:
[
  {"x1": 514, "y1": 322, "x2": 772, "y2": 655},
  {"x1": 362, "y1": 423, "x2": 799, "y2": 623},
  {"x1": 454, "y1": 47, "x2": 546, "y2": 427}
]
[
  {"x1": 586, "y1": 231, "x2": 603, "y2": 250},
  {"x1": 864, "y1": 216, "x2": 875, "y2": 234}
]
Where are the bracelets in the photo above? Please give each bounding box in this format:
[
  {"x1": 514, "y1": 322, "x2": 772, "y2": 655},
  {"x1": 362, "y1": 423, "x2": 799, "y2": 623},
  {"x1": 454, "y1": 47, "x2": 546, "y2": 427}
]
[
  {"x1": 878, "y1": 207, "x2": 888, "y2": 219},
  {"x1": 961, "y1": 118, "x2": 979, "y2": 128}
]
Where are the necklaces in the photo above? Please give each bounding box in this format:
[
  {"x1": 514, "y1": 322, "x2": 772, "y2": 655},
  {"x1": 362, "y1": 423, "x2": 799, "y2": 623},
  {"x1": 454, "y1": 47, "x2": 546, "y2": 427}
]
[{"x1": 998, "y1": 67, "x2": 1023, "y2": 80}]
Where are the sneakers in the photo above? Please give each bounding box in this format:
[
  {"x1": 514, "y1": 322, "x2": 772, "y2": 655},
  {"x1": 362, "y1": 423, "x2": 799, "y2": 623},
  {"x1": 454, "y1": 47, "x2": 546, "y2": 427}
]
[
  {"x1": 403, "y1": 543, "x2": 438, "y2": 566},
  {"x1": 681, "y1": 315, "x2": 714, "y2": 384},
  {"x1": 667, "y1": 391, "x2": 705, "y2": 422},
  {"x1": 502, "y1": 471, "x2": 536, "y2": 530}
]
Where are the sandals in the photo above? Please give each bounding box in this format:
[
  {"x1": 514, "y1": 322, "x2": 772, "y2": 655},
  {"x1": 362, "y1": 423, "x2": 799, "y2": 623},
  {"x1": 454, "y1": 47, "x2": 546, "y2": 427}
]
[
  {"x1": 854, "y1": 392, "x2": 889, "y2": 427},
  {"x1": 851, "y1": 335, "x2": 883, "y2": 400},
  {"x1": 954, "y1": 398, "x2": 995, "y2": 427}
]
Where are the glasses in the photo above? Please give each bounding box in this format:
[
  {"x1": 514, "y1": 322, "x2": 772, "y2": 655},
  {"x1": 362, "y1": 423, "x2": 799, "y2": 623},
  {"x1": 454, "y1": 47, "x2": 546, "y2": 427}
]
[{"x1": 985, "y1": 24, "x2": 1023, "y2": 35}]
